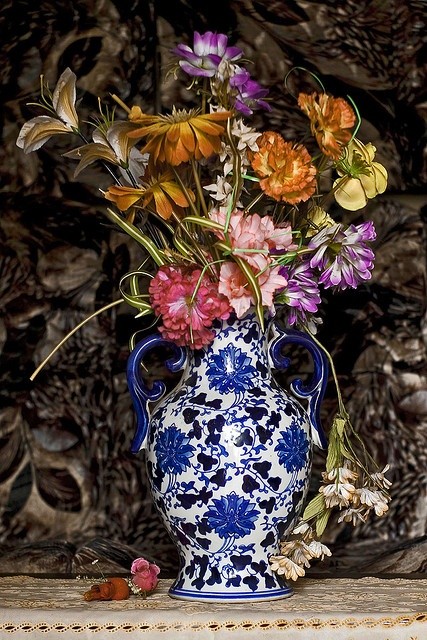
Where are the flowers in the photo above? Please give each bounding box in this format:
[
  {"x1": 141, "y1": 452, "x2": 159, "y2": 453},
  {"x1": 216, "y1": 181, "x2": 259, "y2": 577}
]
[
  {"x1": 127, "y1": 557, "x2": 163, "y2": 599},
  {"x1": 12, "y1": 30, "x2": 395, "y2": 585}
]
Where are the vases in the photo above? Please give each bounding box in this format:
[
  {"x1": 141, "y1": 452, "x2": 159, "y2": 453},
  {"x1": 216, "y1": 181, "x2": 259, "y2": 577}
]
[{"x1": 124, "y1": 315, "x2": 330, "y2": 604}]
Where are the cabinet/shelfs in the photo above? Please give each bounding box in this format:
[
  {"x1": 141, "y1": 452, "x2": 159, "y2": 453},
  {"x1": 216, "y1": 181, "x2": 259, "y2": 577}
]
[{"x1": 0, "y1": 570, "x2": 426, "y2": 640}]
